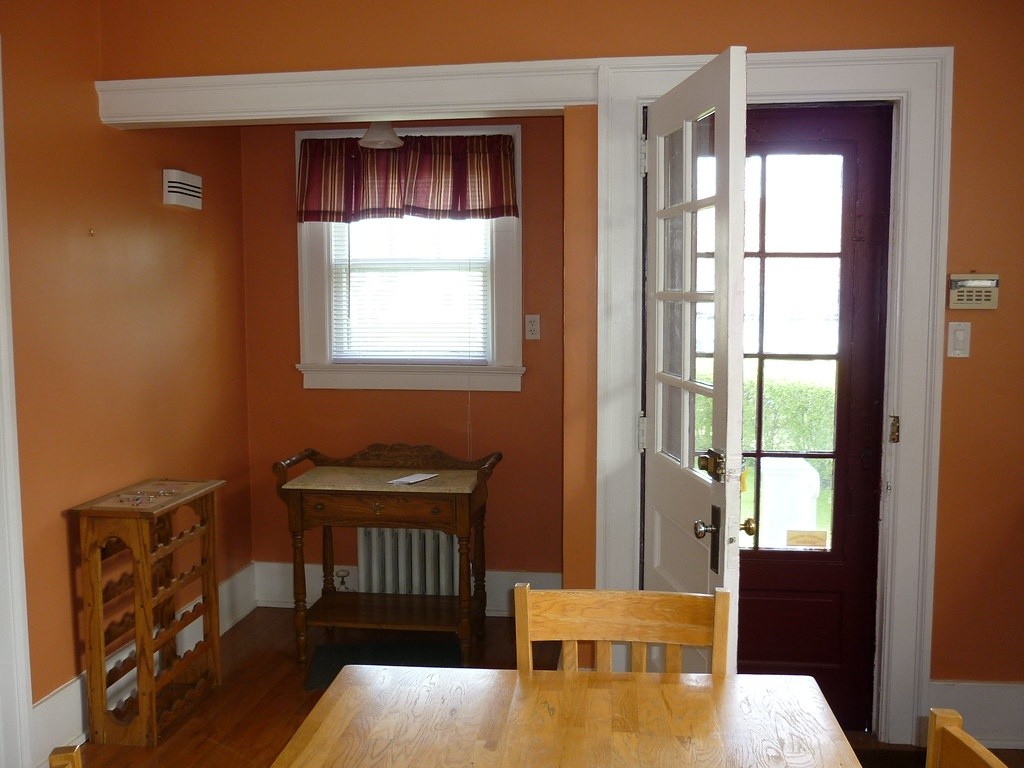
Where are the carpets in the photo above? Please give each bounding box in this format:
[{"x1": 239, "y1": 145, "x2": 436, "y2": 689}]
[{"x1": 303, "y1": 639, "x2": 461, "y2": 690}]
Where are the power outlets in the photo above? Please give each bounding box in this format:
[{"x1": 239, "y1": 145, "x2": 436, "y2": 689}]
[{"x1": 524, "y1": 314, "x2": 541, "y2": 340}]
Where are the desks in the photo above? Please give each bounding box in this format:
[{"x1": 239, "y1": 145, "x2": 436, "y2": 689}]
[
  {"x1": 269, "y1": 664, "x2": 863, "y2": 768},
  {"x1": 67, "y1": 477, "x2": 227, "y2": 749},
  {"x1": 272, "y1": 442, "x2": 504, "y2": 667}
]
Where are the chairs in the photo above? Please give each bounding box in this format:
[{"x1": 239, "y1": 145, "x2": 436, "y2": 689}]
[
  {"x1": 514, "y1": 583, "x2": 731, "y2": 674},
  {"x1": 925, "y1": 707, "x2": 1008, "y2": 768}
]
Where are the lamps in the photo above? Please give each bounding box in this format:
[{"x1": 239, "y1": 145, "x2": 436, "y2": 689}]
[{"x1": 358, "y1": 122, "x2": 404, "y2": 149}]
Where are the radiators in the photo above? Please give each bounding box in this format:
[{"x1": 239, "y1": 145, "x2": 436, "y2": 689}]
[{"x1": 357, "y1": 527, "x2": 475, "y2": 596}]
